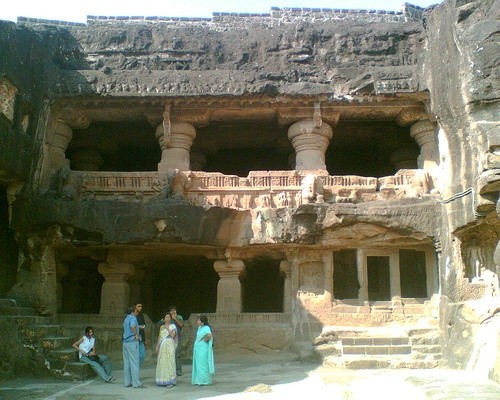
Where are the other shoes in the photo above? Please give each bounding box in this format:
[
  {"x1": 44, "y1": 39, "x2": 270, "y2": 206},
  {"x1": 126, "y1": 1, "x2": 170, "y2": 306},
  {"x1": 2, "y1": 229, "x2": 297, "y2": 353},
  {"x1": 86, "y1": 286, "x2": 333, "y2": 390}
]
[{"x1": 107, "y1": 377, "x2": 116, "y2": 383}]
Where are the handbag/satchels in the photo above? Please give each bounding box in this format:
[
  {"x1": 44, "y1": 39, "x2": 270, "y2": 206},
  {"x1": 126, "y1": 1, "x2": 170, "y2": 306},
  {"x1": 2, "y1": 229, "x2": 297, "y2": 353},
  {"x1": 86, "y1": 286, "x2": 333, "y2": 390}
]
[{"x1": 85, "y1": 348, "x2": 99, "y2": 361}]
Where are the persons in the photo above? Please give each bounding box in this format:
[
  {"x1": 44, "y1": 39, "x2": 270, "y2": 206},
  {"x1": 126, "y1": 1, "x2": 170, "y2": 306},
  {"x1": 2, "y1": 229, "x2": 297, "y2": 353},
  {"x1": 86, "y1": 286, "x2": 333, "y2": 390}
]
[
  {"x1": 72, "y1": 326, "x2": 115, "y2": 383},
  {"x1": 160, "y1": 305, "x2": 184, "y2": 376},
  {"x1": 154, "y1": 312, "x2": 177, "y2": 388},
  {"x1": 122, "y1": 307, "x2": 144, "y2": 388},
  {"x1": 134, "y1": 301, "x2": 146, "y2": 385},
  {"x1": 192, "y1": 316, "x2": 216, "y2": 385}
]
